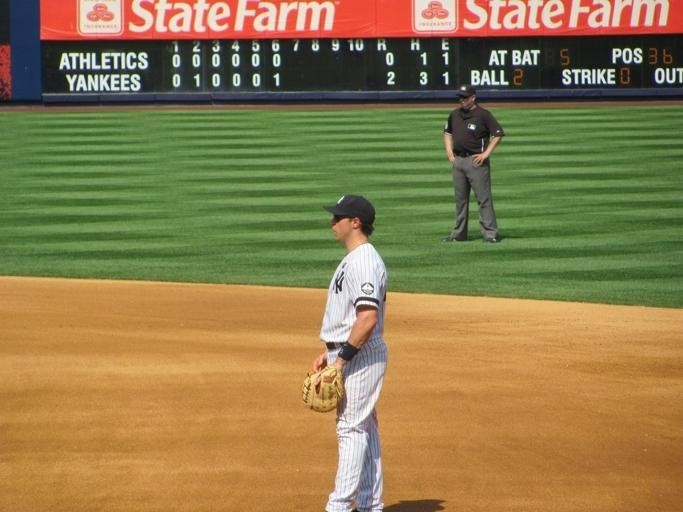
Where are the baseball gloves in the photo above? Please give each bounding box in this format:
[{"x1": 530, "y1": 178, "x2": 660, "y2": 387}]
[{"x1": 302, "y1": 363, "x2": 343, "y2": 412}]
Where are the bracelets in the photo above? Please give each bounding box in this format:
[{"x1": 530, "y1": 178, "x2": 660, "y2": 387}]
[{"x1": 336, "y1": 340, "x2": 360, "y2": 362}]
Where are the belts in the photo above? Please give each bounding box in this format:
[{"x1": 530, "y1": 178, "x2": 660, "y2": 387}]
[
  {"x1": 326, "y1": 342, "x2": 346, "y2": 349},
  {"x1": 455, "y1": 150, "x2": 476, "y2": 158}
]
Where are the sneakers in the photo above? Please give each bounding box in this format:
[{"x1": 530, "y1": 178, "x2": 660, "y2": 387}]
[{"x1": 441, "y1": 234, "x2": 500, "y2": 243}]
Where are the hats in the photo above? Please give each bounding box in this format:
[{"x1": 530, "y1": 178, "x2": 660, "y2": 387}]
[
  {"x1": 322, "y1": 193, "x2": 376, "y2": 227},
  {"x1": 455, "y1": 84, "x2": 477, "y2": 97}
]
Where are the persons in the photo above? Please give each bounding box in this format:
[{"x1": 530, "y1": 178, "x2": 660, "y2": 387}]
[
  {"x1": 300, "y1": 193, "x2": 388, "y2": 512},
  {"x1": 440, "y1": 86, "x2": 504, "y2": 243}
]
